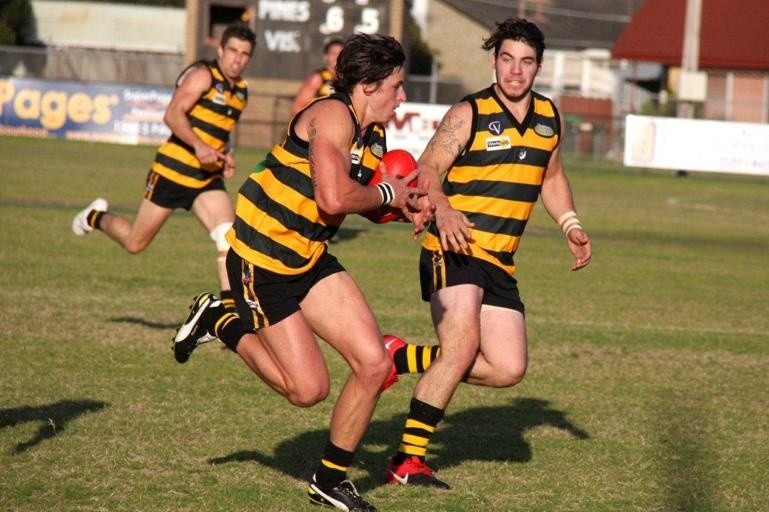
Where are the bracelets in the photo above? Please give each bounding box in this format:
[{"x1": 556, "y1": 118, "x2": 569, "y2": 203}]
[
  {"x1": 557, "y1": 211, "x2": 583, "y2": 239},
  {"x1": 376, "y1": 182, "x2": 395, "y2": 207}
]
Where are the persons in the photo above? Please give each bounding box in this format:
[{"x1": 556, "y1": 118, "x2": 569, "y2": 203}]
[
  {"x1": 72, "y1": 25, "x2": 241, "y2": 320},
  {"x1": 291, "y1": 37, "x2": 345, "y2": 117},
  {"x1": 170, "y1": 33, "x2": 434, "y2": 512},
  {"x1": 382, "y1": 17, "x2": 592, "y2": 489}
]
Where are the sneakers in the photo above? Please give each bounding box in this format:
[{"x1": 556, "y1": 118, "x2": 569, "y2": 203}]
[
  {"x1": 374, "y1": 331, "x2": 409, "y2": 398},
  {"x1": 170, "y1": 289, "x2": 221, "y2": 364},
  {"x1": 384, "y1": 454, "x2": 449, "y2": 492},
  {"x1": 306, "y1": 473, "x2": 381, "y2": 512},
  {"x1": 71, "y1": 196, "x2": 109, "y2": 237}
]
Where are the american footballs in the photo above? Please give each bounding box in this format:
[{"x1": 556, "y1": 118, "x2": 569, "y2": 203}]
[{"x1": 365, "y1": 149, "x2": 418, "y2": 224}]
